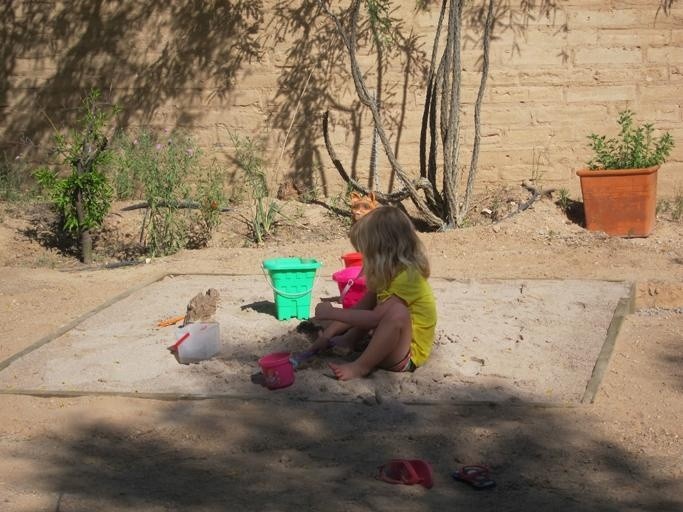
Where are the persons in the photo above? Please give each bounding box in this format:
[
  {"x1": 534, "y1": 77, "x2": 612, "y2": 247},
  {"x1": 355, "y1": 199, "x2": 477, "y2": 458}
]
[{"x1": 287, "y1": 204, "x2": 439, "y2": 383}]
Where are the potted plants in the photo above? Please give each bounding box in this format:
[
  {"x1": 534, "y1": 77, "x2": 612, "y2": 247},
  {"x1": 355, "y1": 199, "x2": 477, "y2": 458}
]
[{"x1": 575, "y1": 110, "x2": 675, "y2": 238}]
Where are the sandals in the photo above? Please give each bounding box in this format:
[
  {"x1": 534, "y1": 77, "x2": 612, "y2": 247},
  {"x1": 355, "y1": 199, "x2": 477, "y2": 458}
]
[
  {"x1": 379, "y1": 459, "x2": 434, "y2": 490},
  {"x1": 451, "y1": 465, "x2": 496, "y2": 491}
]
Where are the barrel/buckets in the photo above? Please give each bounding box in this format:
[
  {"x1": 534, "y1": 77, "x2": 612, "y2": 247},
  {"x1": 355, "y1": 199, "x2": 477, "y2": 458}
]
[
  {"x1": 332, "y1": 266, "x2": 370, "y2": 310},
  {"x1": 341, "y1": 253, "x2": 364, "y2": 265},
  {"x1": 258, "y1": 351, "x2": 298, "y2": 390},
  {"x1": 173, "y1": 323, "x2": 220, "y2": 364},
  {"x1": 261, "y1": 256, "x2": 323, "y2": 320}
]
